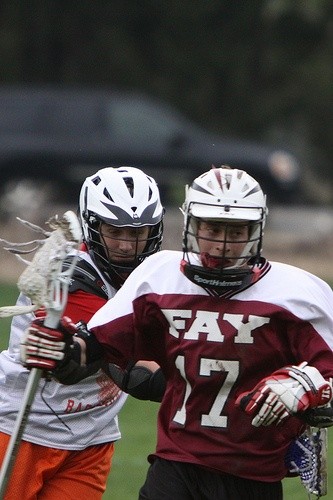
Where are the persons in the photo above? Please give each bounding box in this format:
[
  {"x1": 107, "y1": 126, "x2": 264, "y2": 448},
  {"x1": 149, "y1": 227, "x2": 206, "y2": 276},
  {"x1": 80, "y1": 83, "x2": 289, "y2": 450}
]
[
  {"x1": 20, "y1": 167, "x2": 333, "y2": 500},
  {"x1": 0, "y1": 166, "x2": 170, "y2": 500}
]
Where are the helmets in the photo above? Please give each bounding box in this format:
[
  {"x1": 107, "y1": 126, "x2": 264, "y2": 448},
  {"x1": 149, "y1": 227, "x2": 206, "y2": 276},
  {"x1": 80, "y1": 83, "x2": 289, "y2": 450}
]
[
  {"x1": 78, "y1": 167, "x2": 165, "y2": 285},
  {"x1": 178, "y1": 167, "x2": 269, "y2": 289}
]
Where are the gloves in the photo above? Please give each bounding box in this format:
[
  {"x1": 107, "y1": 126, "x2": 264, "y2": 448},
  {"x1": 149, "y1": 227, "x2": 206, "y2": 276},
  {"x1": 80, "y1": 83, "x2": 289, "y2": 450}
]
[
  {"x1": 21, "y1": 317, "x2": 90, "y2": 383},
  {"x1": 233, "y1": 362, "x2": 332, "y2": 428}
]
